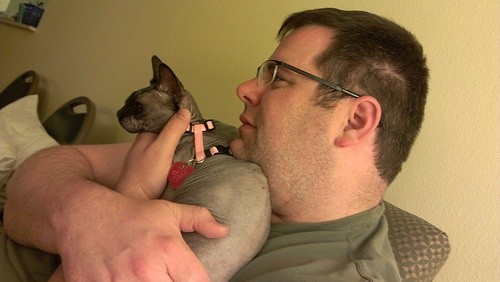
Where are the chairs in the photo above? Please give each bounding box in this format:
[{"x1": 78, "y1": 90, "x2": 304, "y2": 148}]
[{"x1": 200, "y1": 118, "x2": 451, "y2": 282}]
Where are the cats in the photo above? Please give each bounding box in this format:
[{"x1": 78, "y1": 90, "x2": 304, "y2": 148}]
[{"x1": 117, "y1": 54, "x2": 272, "y2": 282}]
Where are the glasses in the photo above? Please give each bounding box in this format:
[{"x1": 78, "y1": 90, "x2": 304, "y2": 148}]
[{"x1": 256, "y1": 59, "x2": 360, "y2": 99}]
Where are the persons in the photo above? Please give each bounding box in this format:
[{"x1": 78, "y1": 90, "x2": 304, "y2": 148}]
[{"x1": 0, "y1": 8, "x2": 430, "y2": 282}]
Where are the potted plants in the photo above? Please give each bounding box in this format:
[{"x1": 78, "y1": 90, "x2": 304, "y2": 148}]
[{"x1": 14, "y1": 1, "x2": 45, "y2": 28}]
